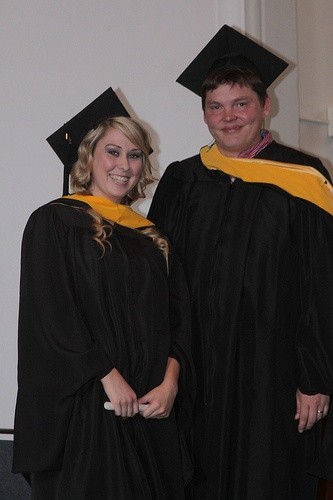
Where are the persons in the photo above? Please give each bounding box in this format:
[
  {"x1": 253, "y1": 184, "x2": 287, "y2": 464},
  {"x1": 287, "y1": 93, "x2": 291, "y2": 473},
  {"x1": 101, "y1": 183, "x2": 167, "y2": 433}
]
[
  {"x1": 12, "y1": 86, "x2": 195, "y2": 500},
  {"x1": 147, "y1": 23, "x2": 333, "y2": 500}
]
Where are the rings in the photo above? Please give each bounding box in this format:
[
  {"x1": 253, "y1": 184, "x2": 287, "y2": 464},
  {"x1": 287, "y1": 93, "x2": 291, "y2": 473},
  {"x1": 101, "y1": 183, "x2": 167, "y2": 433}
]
[{"x1": 317, "y1": 411, "x2": 323, "y2": 413}]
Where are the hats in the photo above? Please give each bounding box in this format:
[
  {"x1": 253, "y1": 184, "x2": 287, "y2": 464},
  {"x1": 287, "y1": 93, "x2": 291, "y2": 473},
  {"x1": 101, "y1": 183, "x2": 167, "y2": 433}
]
[
  {"x1": 175, "y1": 25, "x2": 289, "y2": 98},
  {"x1": 46, "y1": 87, "x2": 154, "y2": 197}
]
